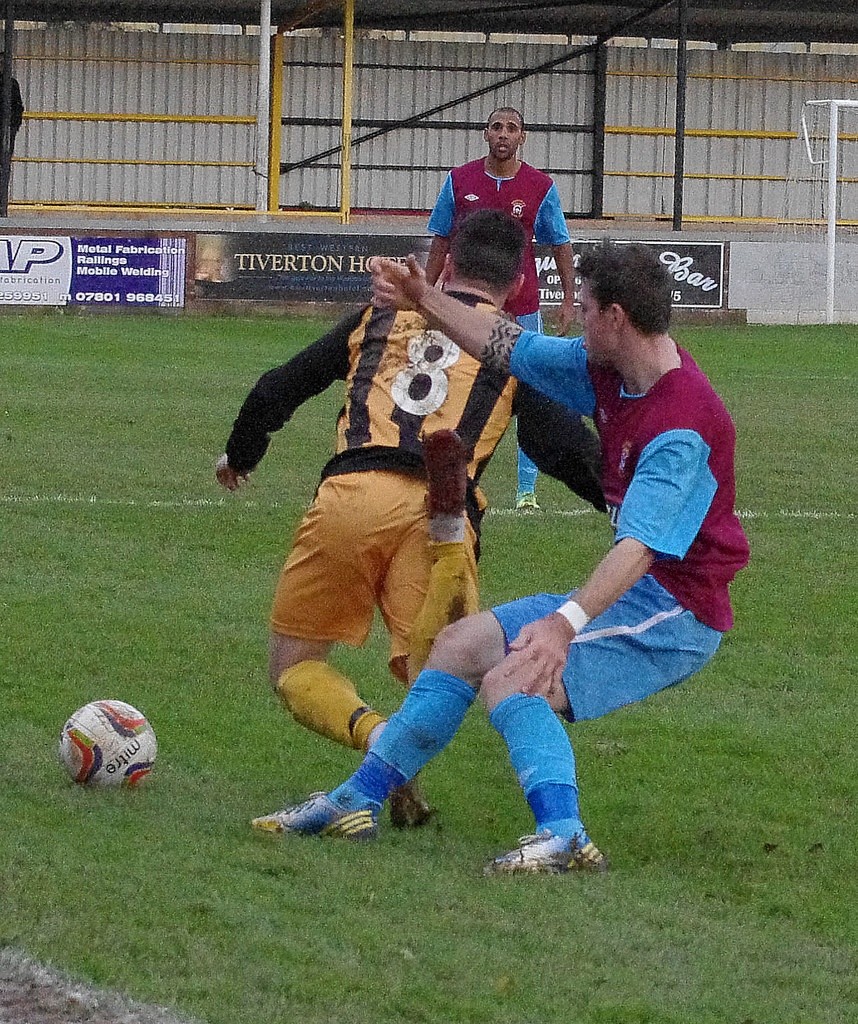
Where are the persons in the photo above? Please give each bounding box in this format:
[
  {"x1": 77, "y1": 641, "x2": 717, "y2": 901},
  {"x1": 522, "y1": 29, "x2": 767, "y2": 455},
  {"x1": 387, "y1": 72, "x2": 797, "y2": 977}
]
[
  {"x1": 213, "y1": 207, "x2": 610, "y2": 829},
  {"x1": 0, "y1": 51, "x2": 25, "y2": 218},
  {"x1": 249, "y1": 237, "x2": 749, "y2": 877},
  {"x1": 424, "y1": 107, "x2": 577, "y2": 508}
]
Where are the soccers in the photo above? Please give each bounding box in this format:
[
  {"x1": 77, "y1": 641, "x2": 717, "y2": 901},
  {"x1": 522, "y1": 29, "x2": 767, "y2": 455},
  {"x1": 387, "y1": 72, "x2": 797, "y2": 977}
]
[{"x1": 60, "y1": 700, "x2": 156, "y2": 792}]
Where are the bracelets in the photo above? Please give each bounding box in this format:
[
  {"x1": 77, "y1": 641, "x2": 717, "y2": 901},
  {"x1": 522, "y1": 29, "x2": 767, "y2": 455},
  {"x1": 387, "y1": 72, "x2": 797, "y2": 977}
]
[{"x1": 556, "y1": 600, "x2": 590, "y2": 635}]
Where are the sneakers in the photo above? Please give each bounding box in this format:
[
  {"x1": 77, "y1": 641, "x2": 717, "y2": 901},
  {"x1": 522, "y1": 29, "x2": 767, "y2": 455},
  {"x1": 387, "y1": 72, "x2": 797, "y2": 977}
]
[
  {"x1": 389, "y1": 774, "x2": 429, "y2": 828},
  {"x1": 483, "y1": 828, "x2": 604, "y2": 875},
  {"x1": 423, "y1": 427, "x2": 468, "y2": 520},
  {"x1": 516, "y1": 493, "x2": 540, "y2": 512},
  {"x1": 248, "y1": 791, "x2": 382, "y2": 843}
]
